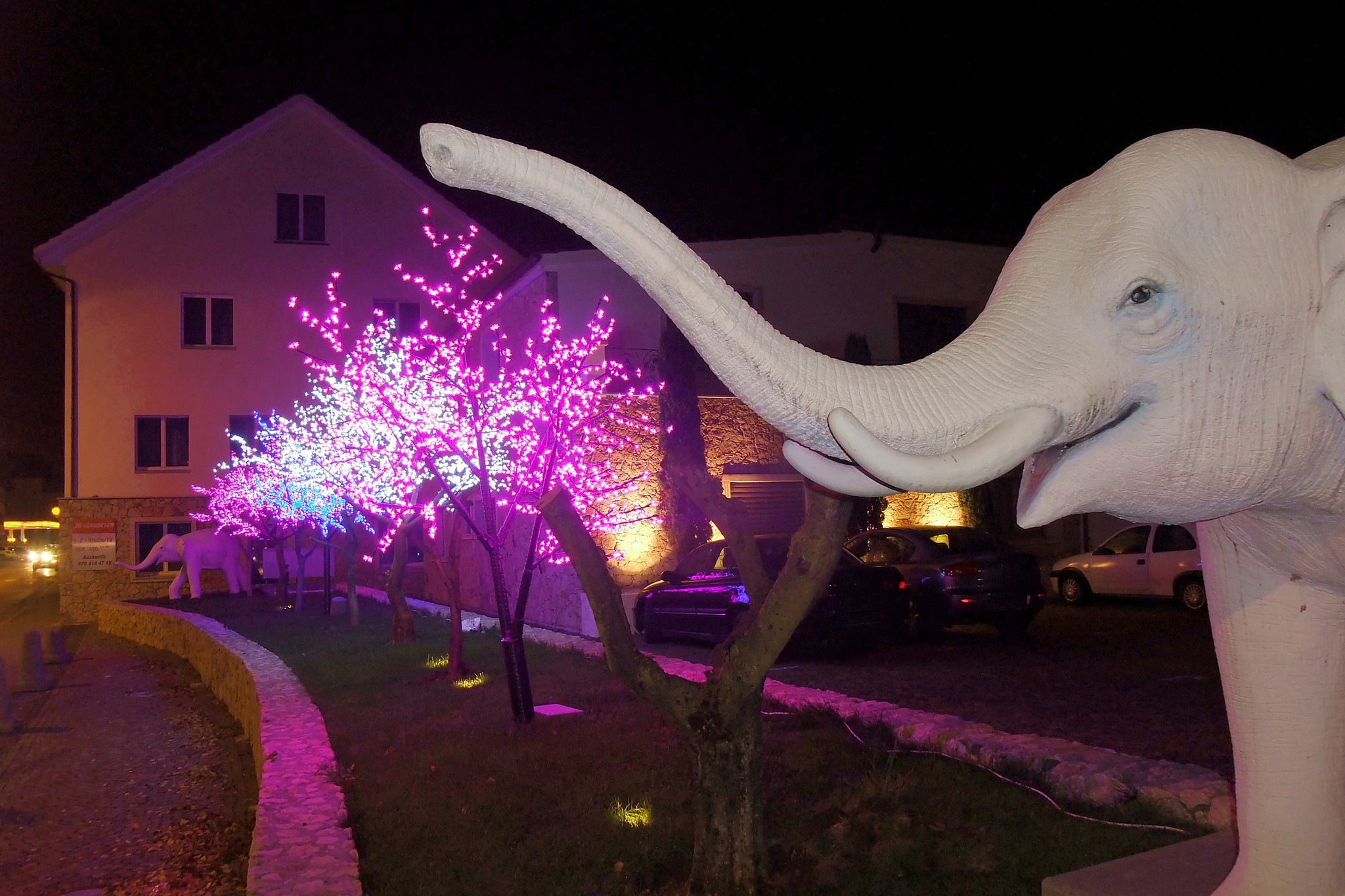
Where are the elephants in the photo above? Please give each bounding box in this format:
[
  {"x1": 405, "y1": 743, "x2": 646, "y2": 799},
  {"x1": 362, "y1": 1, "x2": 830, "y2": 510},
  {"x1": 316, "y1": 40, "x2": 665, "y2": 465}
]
[
  {"x1": 421, "y1": 118, "x2": 1344, "y2": 896},
  {"x1": 116, "y1": 527, "x2": 250, "y2": 600}
]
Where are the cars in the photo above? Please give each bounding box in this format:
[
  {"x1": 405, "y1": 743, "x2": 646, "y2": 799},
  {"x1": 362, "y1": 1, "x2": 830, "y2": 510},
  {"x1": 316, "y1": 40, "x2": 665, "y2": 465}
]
[
  {"x1": 841, "y1": 524, "x2": 1049, "y2": 638},
  {"x1": 1048, "y1": 521, "x2": 1209, "y2": 612},
  {"x1": 0, "y1": 544, "x2": 59, "y2": 572},
  {"x1": 631, "y1": 533, "x2": 911, "y2": 659}
]
